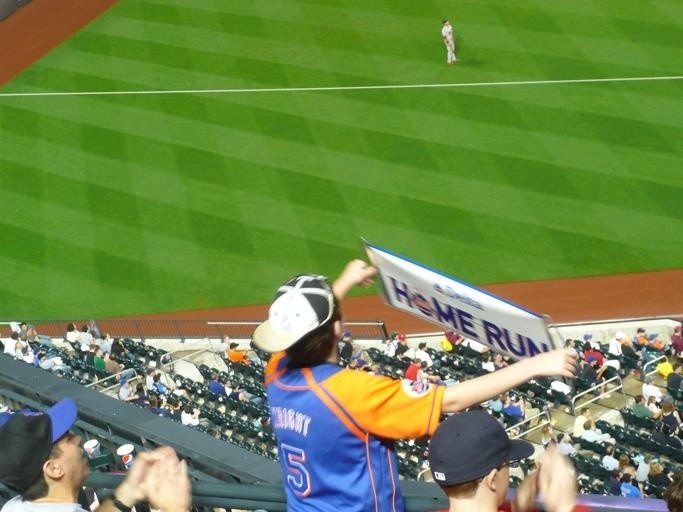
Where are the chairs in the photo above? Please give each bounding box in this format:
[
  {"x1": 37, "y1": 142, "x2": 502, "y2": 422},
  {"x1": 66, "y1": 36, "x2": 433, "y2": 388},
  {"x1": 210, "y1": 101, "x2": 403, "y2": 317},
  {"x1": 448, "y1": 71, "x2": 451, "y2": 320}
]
[{"x1": 0, "y1": 331, "x2": 683, "y2": 512}]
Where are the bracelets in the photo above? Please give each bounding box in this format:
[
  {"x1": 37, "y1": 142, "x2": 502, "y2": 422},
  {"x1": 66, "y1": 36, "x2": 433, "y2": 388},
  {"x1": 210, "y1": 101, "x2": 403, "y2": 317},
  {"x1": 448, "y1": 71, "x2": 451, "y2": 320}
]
[{"x1": 110, "y1": 494, "x2": 131, "y2": 511}]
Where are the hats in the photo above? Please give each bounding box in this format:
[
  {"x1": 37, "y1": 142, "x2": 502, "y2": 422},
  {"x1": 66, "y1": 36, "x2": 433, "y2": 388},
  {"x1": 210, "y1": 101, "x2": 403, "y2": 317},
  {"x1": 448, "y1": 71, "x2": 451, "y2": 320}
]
[
  {"x1": 252, "y1": 277, "x2": 335, "y2": 353},
  {"x1": 429, "y1": 410, "x2": 535, "y2": 486},
  {"x1": 0, "y1": 398, "x2": 79, "y2": 493}
]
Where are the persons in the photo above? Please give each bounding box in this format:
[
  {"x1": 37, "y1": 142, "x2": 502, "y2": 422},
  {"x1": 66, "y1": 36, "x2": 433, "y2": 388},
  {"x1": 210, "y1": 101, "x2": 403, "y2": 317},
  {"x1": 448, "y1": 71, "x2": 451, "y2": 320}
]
[
  {"x1": 428, "y1": 412, "x2": 578, "y2": 511},
  {"x1": 221, "y1": 335, "x2": 261, "y2": 367},
  {"x1": 338, "y1": 328, "x2": 525, "y2": 467},
  {"x1": 1, "y1": 397, "x2": 192, "y2": 511},
  {"x1": 261, "y1": 414, "x2": 272, "y2": 434},
  {"x1": 119, "y1": 368, "x2": 219, "y2": 439},
  {"x1": 208, "y1": 371, "x2": 265, "y2": 404},
  {"x1": 482, "y1": 327, "x2": 682, "y2": 511},
  {"x1": 2, "y1": 321, "x2": 134, "y2": 384},
  {"x1": 440, "y1": 20, "x2": 458, "y2": 65},
  {"x1": 251, "y1": 258, "x2": 578, "y2": 511}
]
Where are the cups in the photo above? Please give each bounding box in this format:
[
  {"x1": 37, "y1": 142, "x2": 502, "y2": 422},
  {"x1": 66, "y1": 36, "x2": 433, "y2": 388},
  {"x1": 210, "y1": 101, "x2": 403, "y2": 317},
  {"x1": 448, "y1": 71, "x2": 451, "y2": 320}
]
[
  {"x1": 83, "y1": 439, "x2": 100, "y2": 458},
  {"x1": 116, "y1": 444, "x2": 137, "y2": 471}
]
